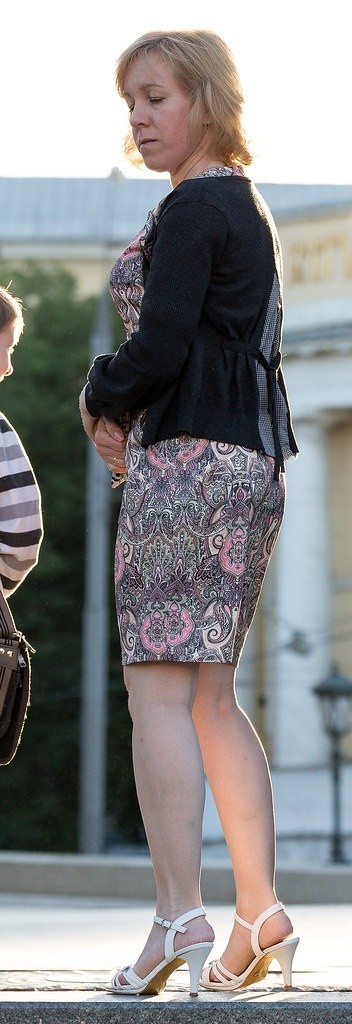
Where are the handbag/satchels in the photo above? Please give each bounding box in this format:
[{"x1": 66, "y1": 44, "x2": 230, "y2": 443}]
[{"x1": 0, "y1": 590, "x2": 36, "y2": 765}]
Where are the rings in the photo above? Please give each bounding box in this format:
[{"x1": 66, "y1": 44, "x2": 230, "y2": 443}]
[{"x1": 112, "y1": 458, "x2": 116, "y2": 465}]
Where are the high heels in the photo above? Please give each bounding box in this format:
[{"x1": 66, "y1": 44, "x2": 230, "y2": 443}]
[
  {"x1": 198, "y1": 902, "x2": 300, "y2": 990},
  {"x1": 106, "y1": 906, "x2": 215, "y2": 996}
]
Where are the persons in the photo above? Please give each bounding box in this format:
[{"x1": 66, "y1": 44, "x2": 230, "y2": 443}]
[
  {"x1": 79, "y1": 30, "x2": 300, "y2": 997},
  {"x1": 0, "y1": 285, "x2": 44, "y2": 598}
]
